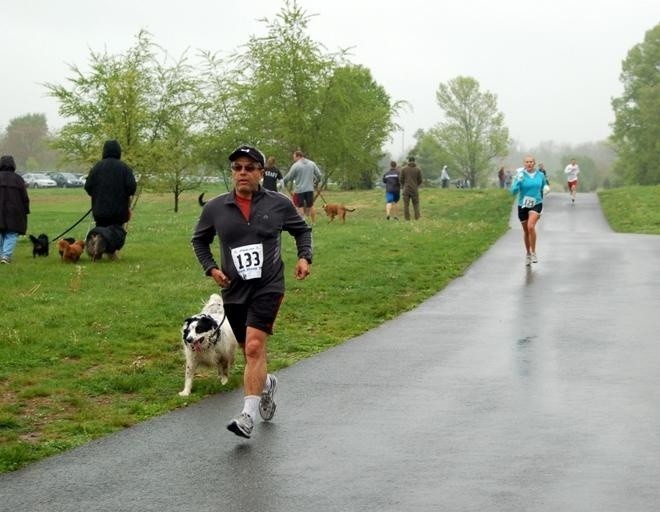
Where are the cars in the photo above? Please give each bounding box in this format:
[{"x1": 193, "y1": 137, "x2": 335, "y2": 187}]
[{"x1": 21, "y1": 171, "x2": 89, "y2": 189}]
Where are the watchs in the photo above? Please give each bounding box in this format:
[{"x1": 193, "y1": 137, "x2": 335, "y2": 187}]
[{"x1": 301, "y1": 254, "x2": 312, "y2": 264}]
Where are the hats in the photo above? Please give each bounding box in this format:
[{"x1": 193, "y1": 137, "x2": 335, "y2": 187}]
[{"x1": 228, "y1": 146, "x2": 266, "y2": 169}]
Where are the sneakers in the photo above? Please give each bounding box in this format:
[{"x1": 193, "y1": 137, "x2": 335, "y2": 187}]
[
  {"x1": 394, "y1": 217, "x2": 399, "y2": 220},
  {"x1": 227, "y1": 413, "x2": 254, "y2": 439},
  {"x1": 572, "y1": 199, "x2": 574, "y2": 202},
  {"x1": 532, "y1": 254, "x2": 537, "y2": 263},
  {"x1": 2, "y1": 256, "x2": 11, "y2": 264},
  {"x1": 259, "y1": 374, "x2": 277, "y2": 421},
  {"x1": 525, "y1": 254, "x2": 531, "y2": 266},
  {"x1": 386, "y1": 216, "x2": 390, "y2": 220}
]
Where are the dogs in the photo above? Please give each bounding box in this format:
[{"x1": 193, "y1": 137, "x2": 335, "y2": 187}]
[
  {"x1": 30, "y1": 232, "x2": 49, "y2": 258},
  {"x1": 86, "y1": 225, "x2": 128, "y2": 263},
  {"x1": 59, "y1": 238, "x2": 75, "y2": 257},
  {"x1": 177, "y1": 294, "x2": 238, "y2": 396},
  {"x1": 322, "y1": 204, "x2": 356, "y2": 224},
  {"x1": 58, "y1": 240, "x2": 85, "y2": 262}
]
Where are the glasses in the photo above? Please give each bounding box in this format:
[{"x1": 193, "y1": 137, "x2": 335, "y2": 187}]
[{"x1": 231, "y1": 164, "x2": 259, "y2": 171}]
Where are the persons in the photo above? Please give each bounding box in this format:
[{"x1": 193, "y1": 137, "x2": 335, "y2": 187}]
[
  {"x1": 498, "y1": 165, "x2": 506, "y2": 188},
  {"x1": 83, "y1": 140, "x2": 137, "y2": 258},
  {"x1": 441, "y1": 164, "x2": 450, "y2": 188},
  {"x1": 0, "y1": 156, "x2": 30, "y2": 265},
  {"x1": 538, "y1": 163, "x2": 550, "y2": 197},
  {"x1": 262, "y1": 156, "x2": 283, "y2": 192},
  {"x1": 278, "y1": 151, "x2": 323, "y2": 217},
  {"x1": 400, "y1": 157, "x2": 423, "y2": 219},
  {"x1": 455, "y1": 179, "x2": 471, "y2": 188},
  {"x1": 383, "y1": 161, "x2": 399, "y2": 221},
  {"x1": 510, "y1": 156, "x2": 550, "y2": 266},
  {"x1": 193, "y1": 145, "x2": 314, "y2": 438},
  {"x1": 505, "y1": 170, "x2": 512, "y2": 187},
  {"x1": 564, "y1": 158, "x2": 580, "y2": 203}
]
